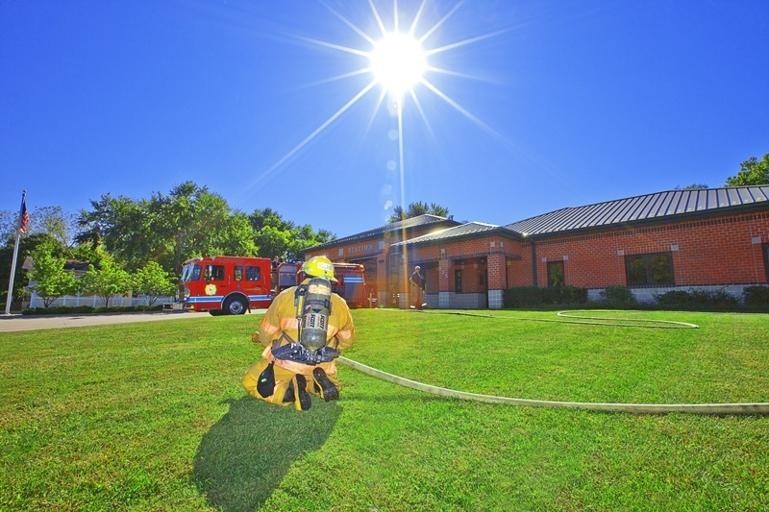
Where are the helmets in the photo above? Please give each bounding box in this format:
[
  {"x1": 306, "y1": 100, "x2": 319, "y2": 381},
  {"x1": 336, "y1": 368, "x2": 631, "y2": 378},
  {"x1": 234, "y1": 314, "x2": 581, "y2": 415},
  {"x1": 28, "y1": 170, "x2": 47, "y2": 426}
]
[{"x1": 297, "y1": 256, "x2": 334, "y2": 281}]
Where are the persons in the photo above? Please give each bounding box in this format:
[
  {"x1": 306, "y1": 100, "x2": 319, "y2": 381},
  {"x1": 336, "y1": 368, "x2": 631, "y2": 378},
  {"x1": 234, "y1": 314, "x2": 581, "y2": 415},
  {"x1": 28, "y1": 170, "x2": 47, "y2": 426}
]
[
  {"x1": 409, "y1": 265, "x2": 425, "y2": 311},
  {"x1": 240, "y1": 255, "x2": 357, "y2": 412}
]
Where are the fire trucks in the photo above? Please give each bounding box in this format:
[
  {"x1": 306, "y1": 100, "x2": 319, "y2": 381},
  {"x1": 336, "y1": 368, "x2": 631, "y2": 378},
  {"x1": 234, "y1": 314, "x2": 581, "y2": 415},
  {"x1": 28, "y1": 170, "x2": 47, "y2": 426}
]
[{"x1": 171, "y1": 256, "x2": 367, "y2": 314}]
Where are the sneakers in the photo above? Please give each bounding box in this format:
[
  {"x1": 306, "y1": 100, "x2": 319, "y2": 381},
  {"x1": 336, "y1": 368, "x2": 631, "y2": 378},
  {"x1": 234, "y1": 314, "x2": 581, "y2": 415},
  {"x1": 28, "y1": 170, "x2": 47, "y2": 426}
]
[
  {"x1": 312, "y1": 366, "x2": 339, "y2": 402},
  {"x1": 294, "y1": 374, "x2": 311, "y2": 412}
]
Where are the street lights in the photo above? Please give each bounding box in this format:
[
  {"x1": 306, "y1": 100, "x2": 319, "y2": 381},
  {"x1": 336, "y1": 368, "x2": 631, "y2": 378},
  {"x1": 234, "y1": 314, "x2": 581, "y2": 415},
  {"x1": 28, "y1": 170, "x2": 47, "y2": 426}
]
[{"x1": 384, "y1": 77, "x2": 409, "y2": 303}]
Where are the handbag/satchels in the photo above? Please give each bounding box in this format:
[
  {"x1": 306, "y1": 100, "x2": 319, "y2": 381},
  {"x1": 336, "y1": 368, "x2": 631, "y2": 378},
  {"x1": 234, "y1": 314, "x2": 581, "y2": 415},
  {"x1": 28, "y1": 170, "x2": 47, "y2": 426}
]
[{"x1": 257, "y1": 363, "x2": 275, "y2": 398}]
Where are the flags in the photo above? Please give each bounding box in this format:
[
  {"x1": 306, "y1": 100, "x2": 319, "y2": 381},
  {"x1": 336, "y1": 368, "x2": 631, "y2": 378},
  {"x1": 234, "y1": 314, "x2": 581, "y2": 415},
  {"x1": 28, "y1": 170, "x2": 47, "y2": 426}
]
[{"x1": 18, "y1": 197, "x2": 29, "y2": 237}]
